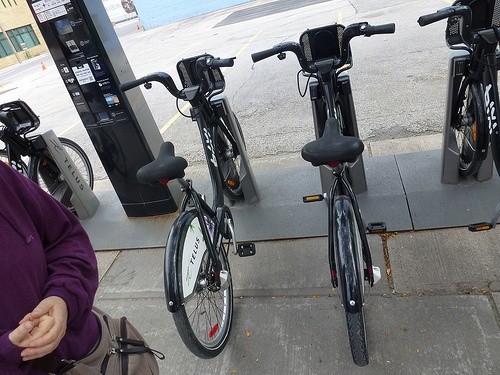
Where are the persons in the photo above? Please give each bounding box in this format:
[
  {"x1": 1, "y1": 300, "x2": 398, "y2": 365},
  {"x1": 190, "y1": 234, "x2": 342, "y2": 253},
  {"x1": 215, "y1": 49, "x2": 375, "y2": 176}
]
[{"x1": 0, "y1": 160, "x2": 101, "y2": 375}]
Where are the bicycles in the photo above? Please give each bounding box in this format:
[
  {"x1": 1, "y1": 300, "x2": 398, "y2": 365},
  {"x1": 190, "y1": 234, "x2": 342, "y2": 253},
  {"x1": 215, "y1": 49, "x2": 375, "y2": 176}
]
[
  {"x1": 251, "y1": 22, "x2": 396, "y2": 367},
  {"x1": 0, "y1": 100, "x2": 94, "y2": 215},
  {"x1": 416, "y1": 0, "x2": 500, "y2": 233},
  {"x1": 118, "y1": 53, "x2": 257, "y2": 360}
]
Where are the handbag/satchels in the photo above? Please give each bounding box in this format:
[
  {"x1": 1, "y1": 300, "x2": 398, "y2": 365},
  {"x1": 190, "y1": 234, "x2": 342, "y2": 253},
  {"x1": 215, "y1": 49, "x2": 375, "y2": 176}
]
[{"x1": 59, "y1": 306, "x2": 161, "y2": 375}]
